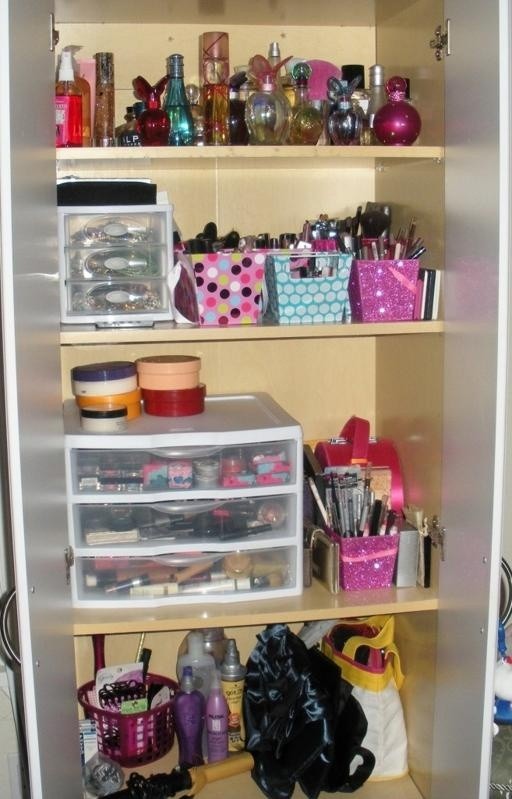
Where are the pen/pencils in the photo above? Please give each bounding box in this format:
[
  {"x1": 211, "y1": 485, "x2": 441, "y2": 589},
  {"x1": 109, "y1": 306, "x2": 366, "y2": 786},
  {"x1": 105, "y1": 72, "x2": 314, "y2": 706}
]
[
  {"x1": 136, "y1": 632, "x2": 145, "y2": 663},
  {"x1": 307, "y1": 461, "x2": 401, "y2": 538},
  {"x1": 352, "y1": 217, "x2": 426, "y2": 260}
]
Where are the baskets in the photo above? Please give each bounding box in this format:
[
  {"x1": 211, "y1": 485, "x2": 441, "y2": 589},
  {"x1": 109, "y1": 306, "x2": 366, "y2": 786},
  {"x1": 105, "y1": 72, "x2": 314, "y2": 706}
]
[{"x1": 78, "y1": 672, "x2": 180, "y2": 767}]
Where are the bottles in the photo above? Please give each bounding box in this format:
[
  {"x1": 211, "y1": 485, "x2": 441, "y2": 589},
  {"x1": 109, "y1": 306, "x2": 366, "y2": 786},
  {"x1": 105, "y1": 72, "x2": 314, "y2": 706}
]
[
  {"x1": 56, "y1": 42, "x2": 422, "y2": 148},
  {"x1": 170, "y1": 626, "x2": 247, "y2": 769}
]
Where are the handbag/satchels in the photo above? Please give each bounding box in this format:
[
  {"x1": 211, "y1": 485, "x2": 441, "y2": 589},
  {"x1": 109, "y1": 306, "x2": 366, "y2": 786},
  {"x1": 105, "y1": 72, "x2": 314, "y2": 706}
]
[{"x1": 243, "y1": 615, "x2": 407, "y2": 799}]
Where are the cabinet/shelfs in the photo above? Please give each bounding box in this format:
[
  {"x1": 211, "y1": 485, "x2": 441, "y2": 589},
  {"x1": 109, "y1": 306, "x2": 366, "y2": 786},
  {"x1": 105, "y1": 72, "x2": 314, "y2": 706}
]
[
  {"x1": 62, "y1": 391, "x2": 304, "y2": 609},
  {"x1": 56, "y1": 177, "x2": 174, "y2": 329},
  {"x1": 0, "y1": 0, "x2": 512, "y2": 799}
]
